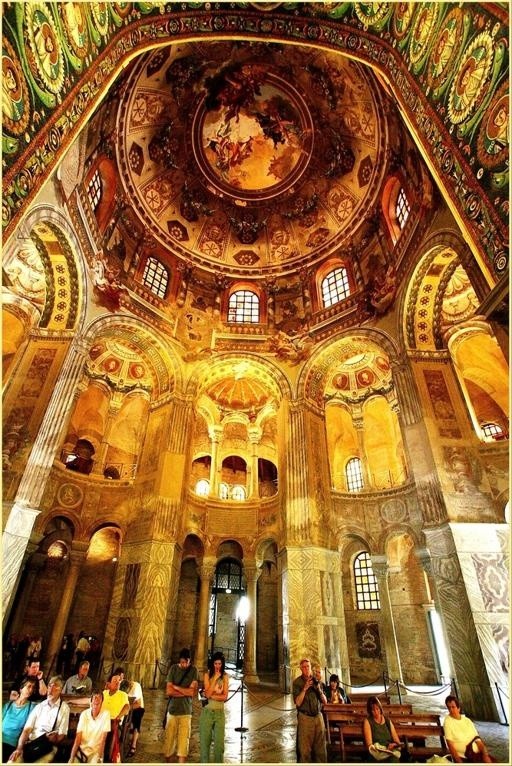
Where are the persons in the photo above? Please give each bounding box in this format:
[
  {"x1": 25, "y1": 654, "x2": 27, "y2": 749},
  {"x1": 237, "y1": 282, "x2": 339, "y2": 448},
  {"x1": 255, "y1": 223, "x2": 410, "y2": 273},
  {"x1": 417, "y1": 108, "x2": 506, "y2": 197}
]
[
  {"x1": 323, "y1": 673, "x2": 353, "y2": 742},
  {"x1": 362, "y1": 696, "x2": 402, "y2": 762},
  {"x1": 198, "y1": 652, "x2": 228, "y2": 762},
  {"x1": 309, "y1": 662, "x2": 324, "y2": 691},
  {"x1": 2, "y1": 630, "x2": 146, "y2": 763},
  {"x1": 443, "y1": 695, "x2": 492, "y2": 763},
  {"x1": 393, "y1": 741, "x2": 399, "y2": 745},
  {"x1": 292, "y1": 659, "x2": 329, "y2": 762},
  {"x1": 163, "y1": 648, "x2": 199, "y2": 763}
]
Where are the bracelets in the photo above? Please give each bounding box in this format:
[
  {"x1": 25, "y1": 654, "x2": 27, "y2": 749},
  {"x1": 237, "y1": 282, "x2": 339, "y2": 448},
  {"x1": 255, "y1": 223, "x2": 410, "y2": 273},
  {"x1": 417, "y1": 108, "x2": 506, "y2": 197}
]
[{"x1": 319, "y1": 689, "x2": 322, "y2": 694}]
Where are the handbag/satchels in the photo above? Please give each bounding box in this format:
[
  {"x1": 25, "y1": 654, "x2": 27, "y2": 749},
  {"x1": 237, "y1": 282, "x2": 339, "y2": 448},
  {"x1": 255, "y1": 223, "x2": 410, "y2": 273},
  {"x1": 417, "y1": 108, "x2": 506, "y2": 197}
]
[
  {"x1": 163, "y1": 716, "x2": 166, "y2": 729},
  {"x1": 427, "y1": 754, "x2": 454, "y2": 762},
  {"x1": 465, "y1": 743, "x2": 481, "y2": 762},
  {"x1": 23, "y1": 732, "x2": 52, "y2": 762}
]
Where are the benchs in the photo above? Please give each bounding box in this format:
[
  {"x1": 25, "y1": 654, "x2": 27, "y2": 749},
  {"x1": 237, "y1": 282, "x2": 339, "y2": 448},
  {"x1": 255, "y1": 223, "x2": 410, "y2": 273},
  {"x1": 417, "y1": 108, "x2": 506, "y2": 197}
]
[
  {"x1": 319, "y1": 693, "x2": 496, "y2": 762},
  {"x1": 2, "y1": 694, "x2": 137, "y2": 763}
]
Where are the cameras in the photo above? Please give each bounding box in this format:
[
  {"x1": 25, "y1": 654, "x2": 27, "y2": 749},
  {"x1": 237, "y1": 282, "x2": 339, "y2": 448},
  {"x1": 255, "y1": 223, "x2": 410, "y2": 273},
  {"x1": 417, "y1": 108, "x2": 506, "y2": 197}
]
[{"x1": 311, "y1": 677, "x2": 319, "y2": 686}]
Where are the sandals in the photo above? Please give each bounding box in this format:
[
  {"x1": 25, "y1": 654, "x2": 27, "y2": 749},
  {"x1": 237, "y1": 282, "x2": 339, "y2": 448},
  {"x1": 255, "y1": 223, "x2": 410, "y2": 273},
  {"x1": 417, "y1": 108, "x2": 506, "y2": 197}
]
[{"x1": 127, "y1": 747, "x2": 136, "y2": 757}]
